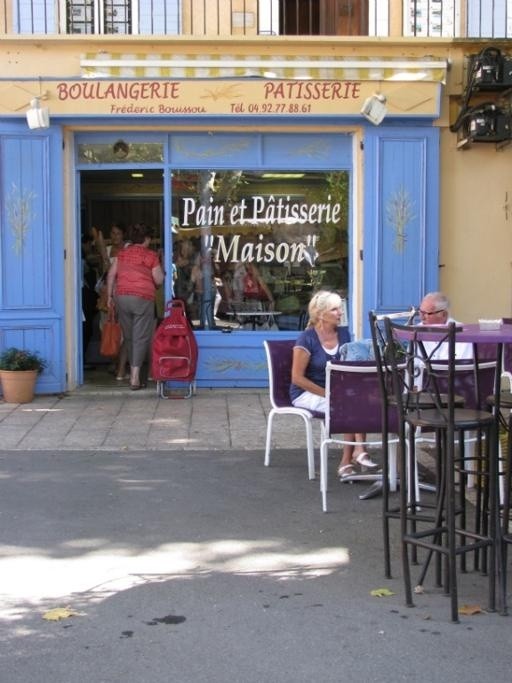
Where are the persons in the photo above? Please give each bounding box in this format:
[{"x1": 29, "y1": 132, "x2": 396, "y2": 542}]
[
  {"x1": 402, "y1": 292, "x2": 473, "y2": 394},
  {"x1": 289, "y1": 291, "x2": 379, "y2": 484},
  {"x1": 91, "y1": 221, "x2": 274, "y2": 391}
]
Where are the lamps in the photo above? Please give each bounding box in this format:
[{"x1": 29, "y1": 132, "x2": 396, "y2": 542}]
[
  {"x1": 361, "y1": 93, "x2": 387, "y2": 125},
  {"x1": 26, "y1": 98, "x2": 50, "y2": 130}
]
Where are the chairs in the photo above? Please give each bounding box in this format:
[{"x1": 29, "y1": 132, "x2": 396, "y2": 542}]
[
  {"x1": 384, "y1": 316, "x2": 502, "y2": 620},
  {"x1": 263, "y1": 338, "x2": 324, "y2": 481},
  {"x1": 369, "y1": 313, "x2": 466, "y2": 576},
  {"x1": 319, "y1": 359, "x2": 411, "y2": 512}
]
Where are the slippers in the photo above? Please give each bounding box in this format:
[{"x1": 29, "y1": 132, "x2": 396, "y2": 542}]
[
  {"x1": 351, "y1": 453, "x2": 378, "y2": 467},
  {"x1": 337, "y1": 465, "x2": 356, "y2": 478}
]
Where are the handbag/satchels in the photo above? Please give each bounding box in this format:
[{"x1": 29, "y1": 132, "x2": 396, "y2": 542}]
[
  {"x1": 339, "y1": 340, "x2": 376, "y2": 361},
  {"x1": 101, "y1": 321, "x2": 120, "y2": 356},
  {"x1": 243, "y1": 272, "x2": 268, "y2": 300},
  {"x1": 152, "y1": 300, "x2": 198, "y2": 382}
]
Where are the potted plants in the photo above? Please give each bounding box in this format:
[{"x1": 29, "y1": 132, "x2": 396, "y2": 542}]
[{"x1": 0, "y1": 347, "x2": 46, "y2": 403}]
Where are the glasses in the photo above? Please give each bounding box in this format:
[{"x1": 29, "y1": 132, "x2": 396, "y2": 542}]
[{"x1": 419, "y1": 309, "x2": 444, "y2": 316}]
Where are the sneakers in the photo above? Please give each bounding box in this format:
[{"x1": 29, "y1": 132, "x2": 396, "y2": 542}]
[
  {"x1": 116, "y1": 373, "x2": 130, "y2": 380},
  {"x1": 130, "y1": 381, "x2": 147, "y2": 390}
]
